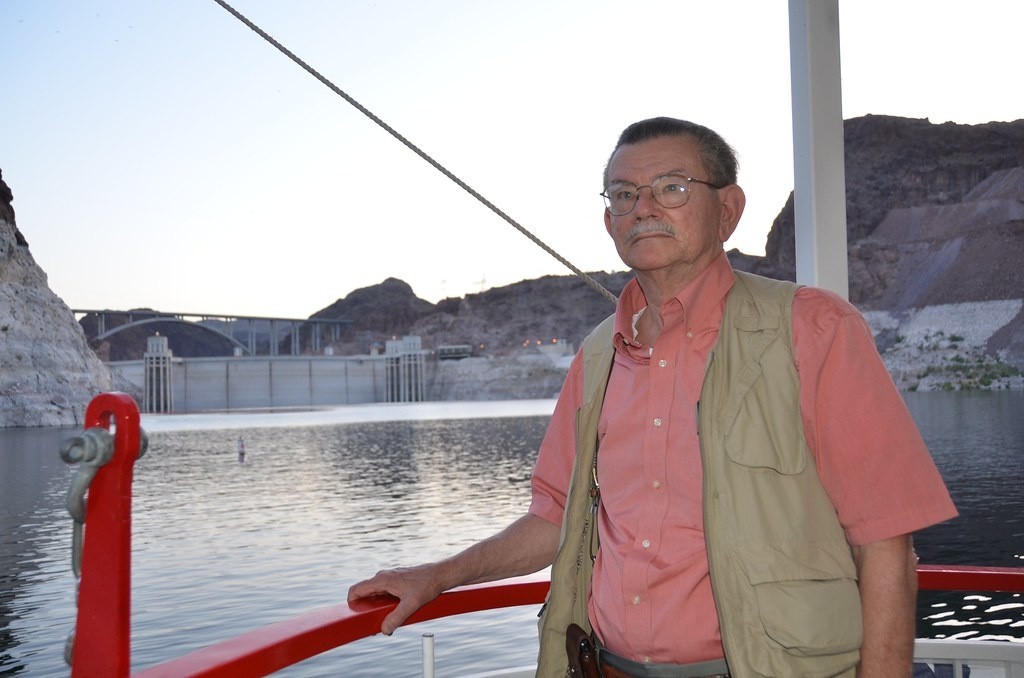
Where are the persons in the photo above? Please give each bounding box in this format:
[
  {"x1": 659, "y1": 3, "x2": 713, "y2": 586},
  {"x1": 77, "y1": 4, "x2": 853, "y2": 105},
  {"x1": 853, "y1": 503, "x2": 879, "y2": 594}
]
[{"x1": 347, "y1": 117, "x2": 960, "y2": 678}]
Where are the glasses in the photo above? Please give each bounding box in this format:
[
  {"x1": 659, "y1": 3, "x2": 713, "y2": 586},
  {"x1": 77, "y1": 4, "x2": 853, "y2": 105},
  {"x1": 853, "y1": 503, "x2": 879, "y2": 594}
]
[{"x1": 602, "y1": 173, "x2": 719, "y2": 216}]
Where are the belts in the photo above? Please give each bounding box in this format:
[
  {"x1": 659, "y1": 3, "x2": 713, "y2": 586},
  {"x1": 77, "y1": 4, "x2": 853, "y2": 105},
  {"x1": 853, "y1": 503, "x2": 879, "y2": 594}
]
[{"x1": 600, "y1": 663, "x2": 730, "y2": 678}]
